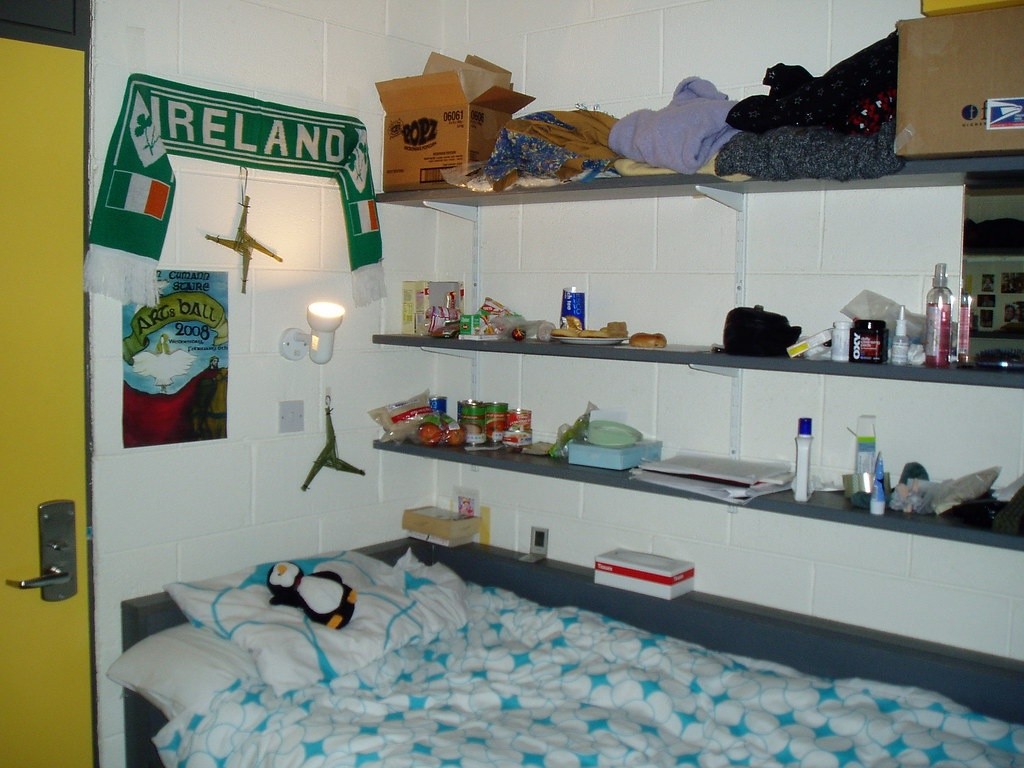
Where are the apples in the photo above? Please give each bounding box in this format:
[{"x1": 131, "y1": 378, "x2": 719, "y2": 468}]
[{"x1": 418, "y1": 423, "x2": 465, "y2": 446}]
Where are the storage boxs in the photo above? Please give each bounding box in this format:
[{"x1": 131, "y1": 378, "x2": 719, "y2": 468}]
[
  {"x1": 401, "y1": 507, "x2": 481, "y2": 548},
  {"x1": 402, "y1": 280, "x2": 460, "y2": 335},
  {"x1": 893, "y1": 0, "x2": 1024, "y2": 159},
  {"x1": 566, "y1": 439, "x2": 664, "y2": 472},
  {"x1": 460, "y1": 314, "x2": 480, "y2": 335},
  {"x1": 592, "y1": 546, "x2": 695, "y2": 600},
  {"x1": 375, "y1": 51, "x2": 536, "y2": 193}
]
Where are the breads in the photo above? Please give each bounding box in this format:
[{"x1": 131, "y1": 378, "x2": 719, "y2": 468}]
[
  {"x1": 551, "y1": 321, "x2": 628, "y2": 338},
  {"x1": 629, "y1": 333, "x2": 667, "y2": 348}
]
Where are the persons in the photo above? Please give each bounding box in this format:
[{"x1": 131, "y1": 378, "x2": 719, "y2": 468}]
[{"x1": 981, "y1": 272, "x2": 1024, "y2": 323}]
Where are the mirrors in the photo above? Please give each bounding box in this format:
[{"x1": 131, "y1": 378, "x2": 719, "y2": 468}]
[{"x1": 961, "y1": 187, "x2": 1024, "y2": 372}]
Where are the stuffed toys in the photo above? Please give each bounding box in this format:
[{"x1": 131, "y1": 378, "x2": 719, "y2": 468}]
[{"x1": 266, "y1": 561, "x2": 358, "y2": 630}]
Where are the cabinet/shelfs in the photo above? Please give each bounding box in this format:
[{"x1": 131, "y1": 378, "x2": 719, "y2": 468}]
[{"x1": 373, "y1": 157, "x2": 1024, "y2": 726}]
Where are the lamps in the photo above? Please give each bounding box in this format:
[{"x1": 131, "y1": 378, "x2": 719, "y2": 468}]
[{"x1": 281, "y1": 302, "x2": 346, "y2": 366}]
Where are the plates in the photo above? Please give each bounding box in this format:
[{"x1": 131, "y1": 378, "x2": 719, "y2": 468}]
[
  {"x1": 585, "y1": 419, "x2": 643, "y2": 448},
  {"x1": 551, "y1": 336, "x2": 630, "y2": 345}
]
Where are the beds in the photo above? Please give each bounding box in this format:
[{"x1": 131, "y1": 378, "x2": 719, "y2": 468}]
[{"x1": 119, "y1": 537, "x2": 1024, "y2": 768}]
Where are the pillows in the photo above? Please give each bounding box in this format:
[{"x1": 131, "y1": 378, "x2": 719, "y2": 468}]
[
  {"x1": 106, "y1": 622, "x2": 255, "y2": 722},
  {"x1": 163, "y1": 550, "x2": 485, "y2": 701}
]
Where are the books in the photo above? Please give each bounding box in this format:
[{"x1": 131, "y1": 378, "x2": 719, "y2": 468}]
[
  {"x1": 628, "y1": 453, "x2": 795, "y2": 506},
  {"x1": 402, "y1": 506, "x2": 482, "y2": 547}
]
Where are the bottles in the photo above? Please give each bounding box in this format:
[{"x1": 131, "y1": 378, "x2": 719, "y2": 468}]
[
  {"x1": 830, "y1": 322, "x2": 850, "y2": 364},
  {"x1": 891, "y1": 305, "x2": 909, "y2": 366},
  {"x1": 869, "y1": 452, "x2": 886, "y2": 515}
]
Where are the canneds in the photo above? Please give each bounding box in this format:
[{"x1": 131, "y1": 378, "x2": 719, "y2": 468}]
[
  {"x1": 428, "y1": 396, "x2": 447, "y2": 413},
  {"x1": 457, "y1": 400, "x2": 532, "y2": 445}
]
